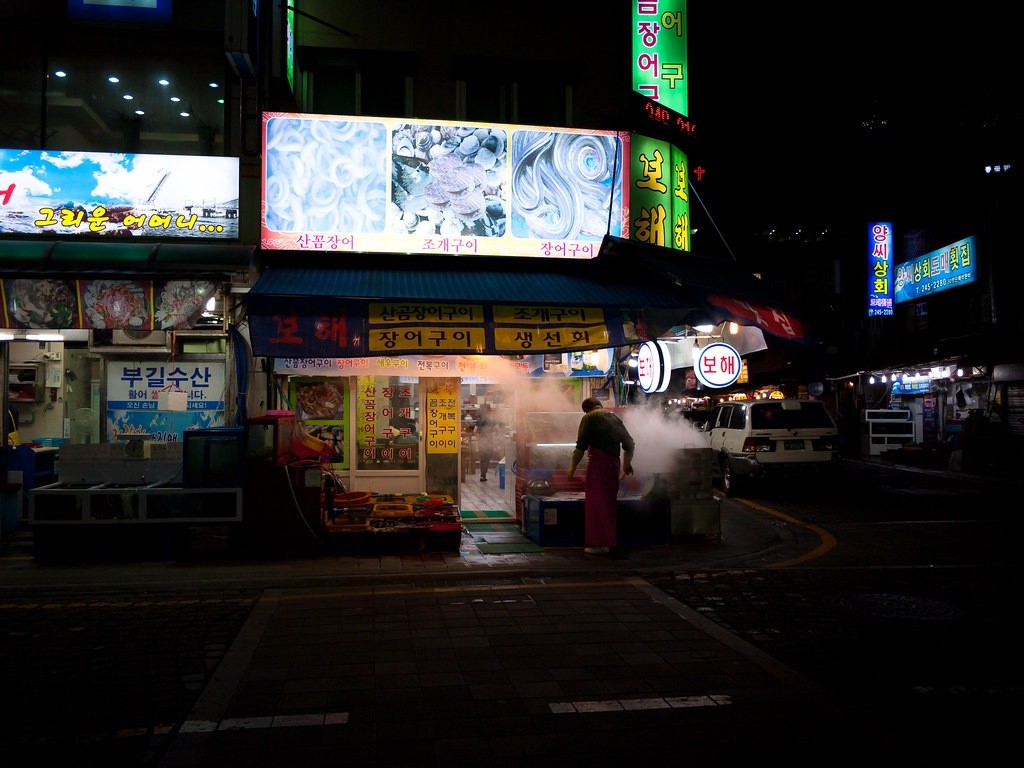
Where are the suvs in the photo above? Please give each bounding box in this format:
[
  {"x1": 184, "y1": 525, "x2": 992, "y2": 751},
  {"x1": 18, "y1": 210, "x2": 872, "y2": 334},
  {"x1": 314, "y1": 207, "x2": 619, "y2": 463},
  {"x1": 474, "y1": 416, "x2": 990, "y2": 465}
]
[{"x1": 706, "y1": 395, "x2": 838, "y2": 492}]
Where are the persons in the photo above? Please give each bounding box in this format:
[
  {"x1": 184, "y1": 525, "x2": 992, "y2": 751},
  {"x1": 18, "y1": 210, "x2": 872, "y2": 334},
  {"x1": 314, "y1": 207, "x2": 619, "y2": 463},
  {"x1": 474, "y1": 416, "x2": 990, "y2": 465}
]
[
  {"x1": 464, "y1": 403, "x2": 503, "y2": 482},
  {"x1": 389, "y1": 417, "x2": 418, "y2": 466},
  {"x1": 567, "y1": 397, "x2": 636, "y2": 557}
]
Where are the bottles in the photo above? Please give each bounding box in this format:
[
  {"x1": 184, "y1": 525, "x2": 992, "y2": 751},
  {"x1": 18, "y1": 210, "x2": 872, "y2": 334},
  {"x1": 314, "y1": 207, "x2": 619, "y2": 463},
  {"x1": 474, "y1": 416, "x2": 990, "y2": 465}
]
[
  {"x1": 583, "y1": 350, "x2": 596, "y2": 370},
  {"x1": 570, "y1": 352, "x2": 583, "y2": 371}
]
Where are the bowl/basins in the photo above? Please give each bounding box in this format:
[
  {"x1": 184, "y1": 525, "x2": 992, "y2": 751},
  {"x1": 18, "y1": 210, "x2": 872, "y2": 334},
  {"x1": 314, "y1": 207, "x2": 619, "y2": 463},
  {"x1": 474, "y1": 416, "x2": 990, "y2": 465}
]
[{"x1": 334, "y1": 491, "x2": 371, "y2": 505}]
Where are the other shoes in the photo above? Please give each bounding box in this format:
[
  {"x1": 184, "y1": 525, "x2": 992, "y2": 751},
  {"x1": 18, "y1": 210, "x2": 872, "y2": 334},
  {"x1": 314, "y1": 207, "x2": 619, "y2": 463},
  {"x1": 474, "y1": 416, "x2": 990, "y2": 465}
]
[{"x1": 585, "y1": 547, "x2": 611, "y2": 554}]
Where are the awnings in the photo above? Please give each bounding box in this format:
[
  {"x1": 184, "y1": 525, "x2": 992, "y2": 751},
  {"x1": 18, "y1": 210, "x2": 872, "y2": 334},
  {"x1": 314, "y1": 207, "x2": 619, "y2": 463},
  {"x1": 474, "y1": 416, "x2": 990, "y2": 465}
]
[
  {"x1": 245, "y1": 267, "x2": 808, "y2": 360},
  {"x1": 827, "y1": 343, "x2": 978, "y2": 382}
]
